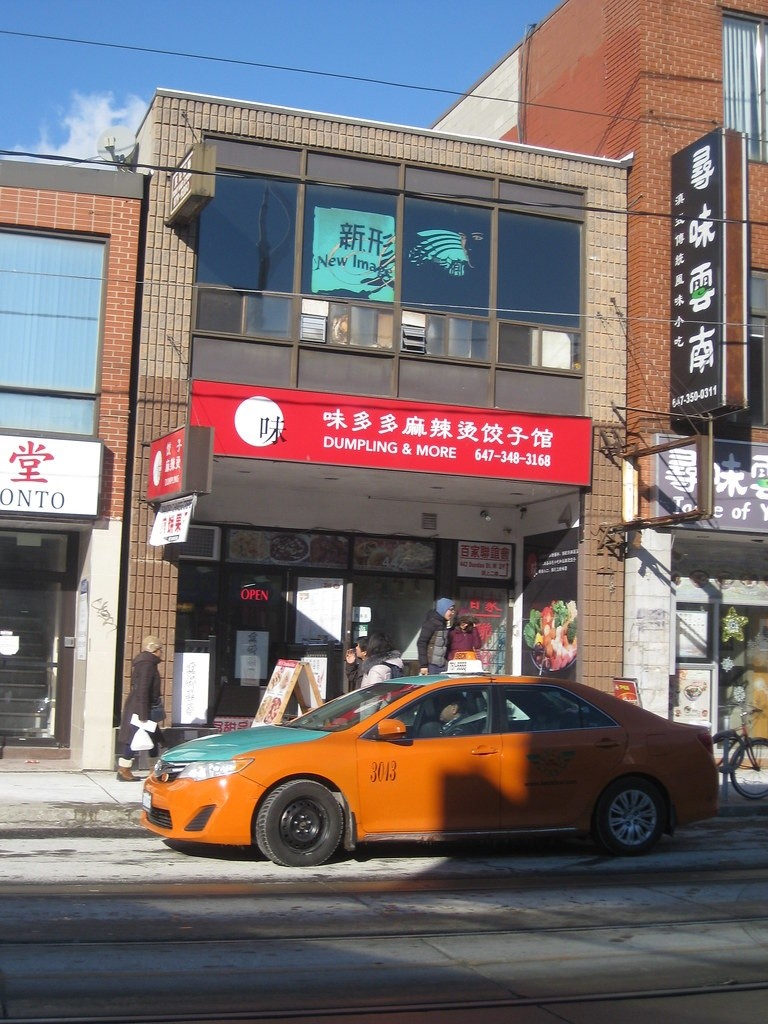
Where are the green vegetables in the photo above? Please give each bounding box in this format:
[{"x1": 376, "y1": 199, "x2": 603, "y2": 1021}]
[{"x1": 523, "y1": 600, "x2": 577, "y2": 649}]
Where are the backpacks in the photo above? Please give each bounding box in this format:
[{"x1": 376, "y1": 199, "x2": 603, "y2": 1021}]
[{"x1": 377, "y1": 661, "x2": 407, "y2": 705}]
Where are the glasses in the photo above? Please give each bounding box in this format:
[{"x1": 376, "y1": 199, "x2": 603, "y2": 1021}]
[{"x1": 448, "y1": 608, "x2": 455, "y2": 611}]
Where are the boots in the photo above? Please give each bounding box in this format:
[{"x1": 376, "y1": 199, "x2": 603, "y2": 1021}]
[{"x1": 116, "y1": 757, "x2": 141, "y2": 780}]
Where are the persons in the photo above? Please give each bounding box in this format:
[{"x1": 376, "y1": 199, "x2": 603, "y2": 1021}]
[
  {"x1": 416, "y1": 597, "x2": 482, "y2": 675},
  {"x1": 437, "y1": 690, "x2": 473, "y2": 737},
  {"x1": 345, "y1": 632, "x2": 403, "y2": 723},
  {"x1": 118, "y1": 635, "x2": 165, "y2": 783}
]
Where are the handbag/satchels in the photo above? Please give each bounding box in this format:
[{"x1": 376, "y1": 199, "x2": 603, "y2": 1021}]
[{"x1": 131, "y1": 727, "x2": 154, "y2": 751}]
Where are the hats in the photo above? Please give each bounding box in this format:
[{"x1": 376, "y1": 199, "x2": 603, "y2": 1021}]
[{"x1": 142, "y1": 635, "x2": 162, "y2": 653}]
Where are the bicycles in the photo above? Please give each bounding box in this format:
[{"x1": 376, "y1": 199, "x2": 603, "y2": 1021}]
[{"x1": 711, "y1": 698, "x2": 768, "y2": 800}]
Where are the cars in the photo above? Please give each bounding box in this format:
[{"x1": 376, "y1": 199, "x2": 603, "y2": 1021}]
[{"x1": 137, "y1": 652, "x2": 719, "y2": 866}]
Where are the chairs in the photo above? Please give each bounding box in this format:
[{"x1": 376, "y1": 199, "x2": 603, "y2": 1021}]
[
  {"x1": 524, "y1": 698, "x2": 561, "y2": 730},
  {"x1": 419, "y1": 698, "x2": 442, "y2": 737},
  {"x1": 465, "y1": 691, "x2": 488, "y2": 731}
]
